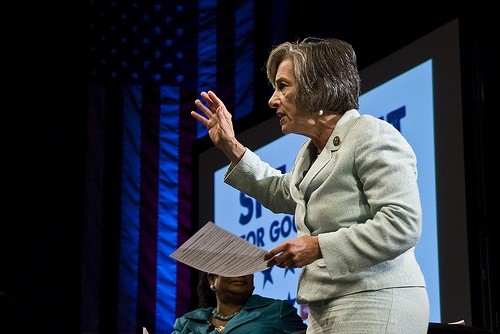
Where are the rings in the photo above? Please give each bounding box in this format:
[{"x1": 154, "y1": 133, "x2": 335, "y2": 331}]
[{"x1": 284, "y1": 261, "x2": 289, "y2": 269}]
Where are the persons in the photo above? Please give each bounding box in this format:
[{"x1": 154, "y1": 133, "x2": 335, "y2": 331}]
[
  {"x1": 171, "y1": 273, "x2": 309, "y2": 334},
  {"x1": 191, "y1": 37, "x2": 430, "y2": 334}
]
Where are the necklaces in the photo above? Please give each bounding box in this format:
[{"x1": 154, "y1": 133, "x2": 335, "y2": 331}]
[{"x1": 212, "y1": 308, "x2": 239, "y2": 320}]
